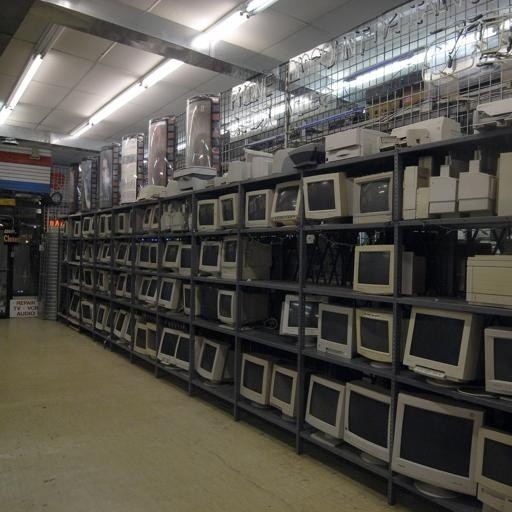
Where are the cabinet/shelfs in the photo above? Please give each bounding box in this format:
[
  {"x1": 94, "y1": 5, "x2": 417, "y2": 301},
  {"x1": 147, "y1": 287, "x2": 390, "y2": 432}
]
[{"x1": 58, "y1": 127, "x2": 510, "y2": 509}]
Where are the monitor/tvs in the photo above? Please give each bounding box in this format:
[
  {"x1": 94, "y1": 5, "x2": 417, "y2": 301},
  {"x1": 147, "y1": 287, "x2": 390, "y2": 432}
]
[
  {"x1": 354, "y1": 172, "x2": 393, "y2": 221},
  {"x1": 217, "y1": 290, "x2": 268, "y2": 330},
  {"x1": 400, "y1": 305, "x2": 476, "y2": 389},
  {"x1": 475, "y1": 426, "x2": 512, "y2": 512},
  {"x1": 484, "y1": 329, "x2": 512, "y2": 399},
  {"x1": 356, "y1": 305, "x2": 407, "y2": 373},
  {"x1": 246, "y1": 189, "x2": 276, "y2": 227},
  {"x1": 240, "y1": 352, "x2": 297, "y2": 422},
  {"x1": 197, "y1": 199, "x2": 224, "y2": 232},
  {"x1": 63, "y1": 201, "x2": 213, "y2": 372},
  {"x1": 304, "y1": 373, "x2": 346, "y2": 448},
  {"x1": 199, "y1": 241, "x2": 222, "y2": 278},
  {"x1": 392, "y1": 393, "x2": 484, "y2": 498},
  {"x1": 280, "y1": 292, "x2": 327, "y2": 350},
  {"x1": 221, "y1": 235, "x2": 272, "y2": 281},
  {"x1": 344, "y1": 380, "x2": 393, "y2": 465},
  {"x1": 197, "y1": 339, "x2": 242, "y2": 386},
  {"x1": 316, "y1": 299, "x2": 355, "y2": 360},
  {"x1": 271, "y1": 180, "x2": 301, "y2": 227},
  {"x1": 303, "y1": 172, "x2": 352, "y2": 220},
  {"x1": 353, "y1": 245, "x2": 396, "y2": 295},
  {"x1": 219, "y1": 193, "x2": 240, "y2": 228}
]
